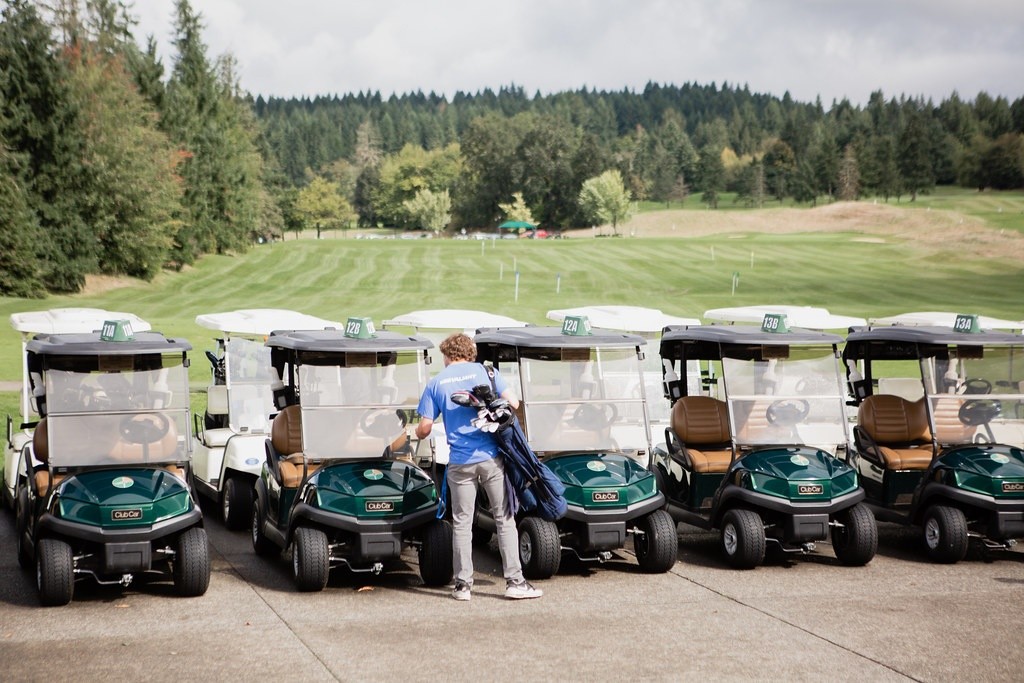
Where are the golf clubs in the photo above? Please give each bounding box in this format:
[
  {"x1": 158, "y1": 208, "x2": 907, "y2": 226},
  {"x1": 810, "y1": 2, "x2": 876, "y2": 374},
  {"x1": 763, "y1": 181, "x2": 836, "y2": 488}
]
[{"x1": 470, "y1": 407, "x2": 512, "y2": 434}]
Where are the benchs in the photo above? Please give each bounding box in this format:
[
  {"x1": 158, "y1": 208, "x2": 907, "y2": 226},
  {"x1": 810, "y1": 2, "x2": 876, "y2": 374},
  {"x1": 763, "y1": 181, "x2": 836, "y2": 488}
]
[
  {"x1": 504, "y1": 397, "x2": 612, "y2": 462},
  {"x1": 854, "y1": 392, "x2": 996, "y2": 469},
  {"x1": 26, "y1": 410, "x2": 178, "y2": 495},
  {"x1": 665, "y1": 395, "x2": 803, "y2": 473},
  {"x1": 265, "y1": 404, "x2": 412, "y2": 487}
]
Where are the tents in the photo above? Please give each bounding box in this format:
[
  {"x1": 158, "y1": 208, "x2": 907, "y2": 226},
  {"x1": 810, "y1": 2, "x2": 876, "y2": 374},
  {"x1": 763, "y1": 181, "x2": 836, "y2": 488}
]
[{"x1": 499, "y1": 221, "x2": 535, "y2": 239}]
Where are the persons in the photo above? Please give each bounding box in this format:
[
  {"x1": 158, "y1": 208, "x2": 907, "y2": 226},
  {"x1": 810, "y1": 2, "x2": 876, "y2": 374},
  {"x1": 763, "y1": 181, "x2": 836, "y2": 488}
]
[{"x1": 416, "y1": 333, "x2": 543, "y2": 600}]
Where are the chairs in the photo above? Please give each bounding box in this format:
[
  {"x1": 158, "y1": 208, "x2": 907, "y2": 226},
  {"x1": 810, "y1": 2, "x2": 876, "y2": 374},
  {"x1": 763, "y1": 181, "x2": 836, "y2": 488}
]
[{"x1": 195, "y1": 385, "x2": 235, "y2": 449}]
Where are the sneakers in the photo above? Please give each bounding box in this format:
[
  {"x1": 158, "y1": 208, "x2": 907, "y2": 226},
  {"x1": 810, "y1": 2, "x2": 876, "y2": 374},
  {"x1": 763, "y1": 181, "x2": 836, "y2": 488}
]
[
  {"x1": 504, "y1": 580, "x2": 543, "y2": 598},
  {"x1": 452, "y1": 582, "x2": 471, "y2": 600}
]
